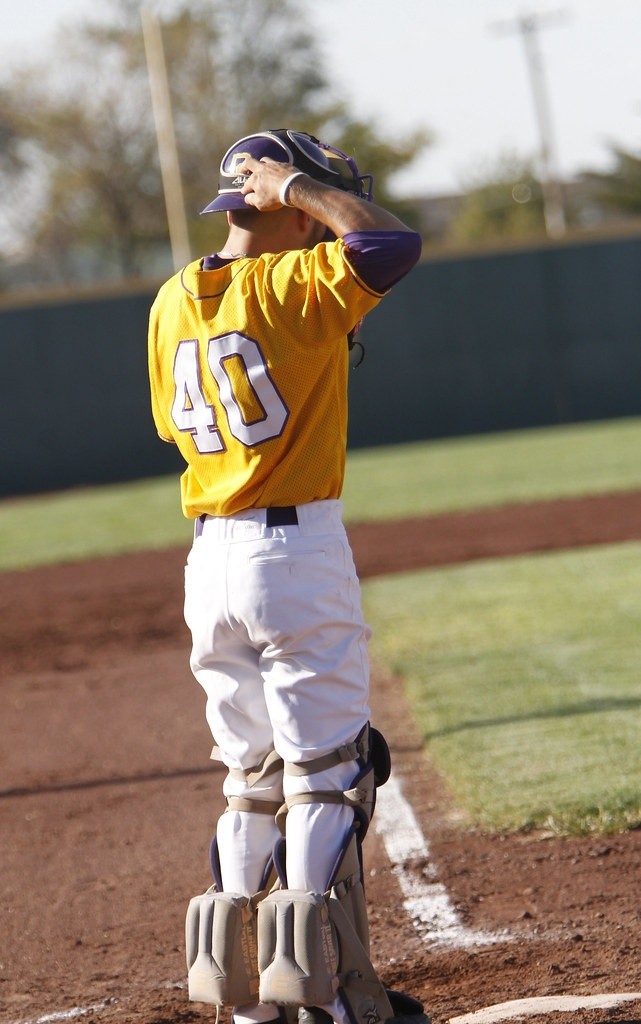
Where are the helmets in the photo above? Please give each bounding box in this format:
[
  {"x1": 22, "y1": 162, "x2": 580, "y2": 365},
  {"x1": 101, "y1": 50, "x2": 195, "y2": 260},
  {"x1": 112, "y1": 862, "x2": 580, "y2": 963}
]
[{"x1": 199, "y1": 129, "x2": 331, "y2": 213}]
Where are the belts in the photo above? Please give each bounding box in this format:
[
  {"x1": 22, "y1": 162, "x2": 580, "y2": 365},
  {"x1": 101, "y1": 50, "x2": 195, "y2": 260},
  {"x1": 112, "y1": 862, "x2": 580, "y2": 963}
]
[{"x1": 195, "y1": 507, "x2": 298, "y2": 536}]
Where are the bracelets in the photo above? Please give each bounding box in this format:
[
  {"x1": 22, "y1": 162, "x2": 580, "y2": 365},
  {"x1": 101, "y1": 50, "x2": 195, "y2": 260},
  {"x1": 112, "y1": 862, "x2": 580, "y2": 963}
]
[{"x1": 279, "y1": 172, "x2": 309, "y2": 208}]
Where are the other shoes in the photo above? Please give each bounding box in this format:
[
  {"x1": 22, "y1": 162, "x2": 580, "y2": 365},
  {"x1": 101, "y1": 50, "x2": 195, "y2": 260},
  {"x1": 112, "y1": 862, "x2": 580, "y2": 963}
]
[{"x1": 298, "y1": 990, "x2": 427, "y2": 1024}]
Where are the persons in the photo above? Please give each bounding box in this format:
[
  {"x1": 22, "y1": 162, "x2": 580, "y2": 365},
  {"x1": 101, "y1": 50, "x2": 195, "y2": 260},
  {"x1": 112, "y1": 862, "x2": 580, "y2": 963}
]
[{"x1": 146, "y1": 130, "x2": 428, "y2": 1024}]
